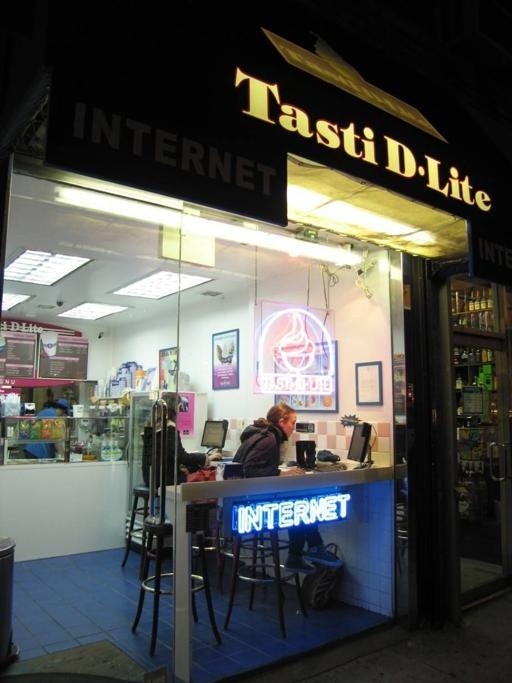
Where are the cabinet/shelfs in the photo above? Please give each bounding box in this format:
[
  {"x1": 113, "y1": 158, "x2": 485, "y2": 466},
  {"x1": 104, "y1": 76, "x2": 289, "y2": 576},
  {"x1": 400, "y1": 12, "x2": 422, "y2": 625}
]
[
  {"x1": 68, "y1": 415, "x2": 130, "y2": 463},
  {"x1": 448, "y1": 281, "x2": 512, "y2": 567},
  {"x1": 1, "y1": 414, "x2": 67, "y2": 465}
]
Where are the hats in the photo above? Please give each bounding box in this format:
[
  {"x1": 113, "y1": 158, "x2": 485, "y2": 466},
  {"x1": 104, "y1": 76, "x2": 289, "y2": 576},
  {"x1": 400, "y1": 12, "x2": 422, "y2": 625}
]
[{"x1": 57, "y1": 398, "x2": 68, "y2": 407}]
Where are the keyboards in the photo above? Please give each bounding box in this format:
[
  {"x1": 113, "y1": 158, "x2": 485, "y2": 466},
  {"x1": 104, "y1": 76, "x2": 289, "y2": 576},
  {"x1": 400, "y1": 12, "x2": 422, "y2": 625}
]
[{"x1": 314, "y1": 463, "x2": 347, "y2": 472}]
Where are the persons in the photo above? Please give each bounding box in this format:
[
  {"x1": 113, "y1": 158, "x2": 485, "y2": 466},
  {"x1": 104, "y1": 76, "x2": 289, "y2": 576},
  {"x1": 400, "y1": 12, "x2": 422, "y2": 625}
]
[
  {"x1": 21, "y1": 395, "x2": 72, "y2": 460},
  {"x1": 141, "y1": 389, "x2": 224, "y2": 490},
  {"x1": 230, "y1": 401, "x2": 345, "y2": 576}
]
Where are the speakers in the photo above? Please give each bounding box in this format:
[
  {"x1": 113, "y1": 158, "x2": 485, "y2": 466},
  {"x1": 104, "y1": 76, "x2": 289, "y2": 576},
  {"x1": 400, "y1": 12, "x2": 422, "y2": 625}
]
[{"x1": 296, "y1": 441, "x2": 316, "y2": 468}]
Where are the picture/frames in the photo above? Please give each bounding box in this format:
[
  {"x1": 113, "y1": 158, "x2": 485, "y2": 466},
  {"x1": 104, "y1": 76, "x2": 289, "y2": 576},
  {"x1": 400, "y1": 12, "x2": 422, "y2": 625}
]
[
  {"x1": 353, "y1": 360, "x2": 384, "y2": 407},
  {"x1": 272, "y1": 339, "x2": 340, "y2": 414},
  {"x1": 158, "y1": 346, "x2": 180, "y2": 393},
  {"x1": 211, "y1": 328, "x2": 241, "y2": 390}
]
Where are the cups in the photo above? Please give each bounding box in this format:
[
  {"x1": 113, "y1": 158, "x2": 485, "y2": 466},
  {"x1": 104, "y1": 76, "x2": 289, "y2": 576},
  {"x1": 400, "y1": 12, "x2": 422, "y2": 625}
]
[{"x1": 41, "y1": 330, "x2": 57, "y2": 357}]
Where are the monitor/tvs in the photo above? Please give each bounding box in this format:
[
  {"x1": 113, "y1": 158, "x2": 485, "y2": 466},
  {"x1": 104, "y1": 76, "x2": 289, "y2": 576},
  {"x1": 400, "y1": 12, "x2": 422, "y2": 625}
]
[
  {"x1": 201, "y1": 420, "x2": 228, "y2": 453},
  {"x1": 347, "y1": 422, "x2": 371, "y2": 469}
]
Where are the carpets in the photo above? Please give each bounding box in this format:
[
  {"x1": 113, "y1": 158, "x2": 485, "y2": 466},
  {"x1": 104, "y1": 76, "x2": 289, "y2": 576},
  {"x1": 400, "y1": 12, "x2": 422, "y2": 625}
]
[{"x1": 1, "y1": 637, "x2": 172, "y2": 681}]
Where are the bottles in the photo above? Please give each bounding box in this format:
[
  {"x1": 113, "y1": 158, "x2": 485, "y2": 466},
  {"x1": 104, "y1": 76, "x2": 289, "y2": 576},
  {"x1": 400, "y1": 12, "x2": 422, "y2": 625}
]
[
  {"x1": 80, "y1": 432, "x2": 121, "y2": 460},
  {"x1": 452, "y1": 345, "x2": 495, "y2": 465},
  {"x1": 450, "y1": 284, "x2": 496, "y2": 334}
]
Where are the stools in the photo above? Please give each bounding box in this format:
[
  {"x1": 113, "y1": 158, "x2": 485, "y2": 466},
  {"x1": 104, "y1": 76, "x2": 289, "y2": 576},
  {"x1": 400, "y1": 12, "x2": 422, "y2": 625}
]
[
  {"x1": 120, "y1": 483, "x2": 168, "y2": 581},
  {"x1": 131, "y1": 513, "x2": 222, "y2": 657},
  {"x1": 224, "y1": 521, "x2": 309, "y2": 640}
]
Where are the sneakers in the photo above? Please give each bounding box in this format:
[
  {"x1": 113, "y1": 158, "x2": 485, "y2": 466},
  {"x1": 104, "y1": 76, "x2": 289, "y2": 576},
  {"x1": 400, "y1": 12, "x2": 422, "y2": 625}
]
[
  {"x1": 285, "y1": 556, "x2": 316, "y2": 573},
  {"x1": 304, "y1": 545, "x2": 342, "y2": 566}
]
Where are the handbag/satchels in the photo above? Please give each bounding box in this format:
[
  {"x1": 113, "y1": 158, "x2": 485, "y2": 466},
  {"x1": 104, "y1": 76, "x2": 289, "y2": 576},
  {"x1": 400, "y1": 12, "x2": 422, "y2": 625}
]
[
  {"x1": 188, "y1": 467, "x2": 218, "y2": 505},
  {"x1": 301, "y1": 543, "x2": 343, "y2": 610}
]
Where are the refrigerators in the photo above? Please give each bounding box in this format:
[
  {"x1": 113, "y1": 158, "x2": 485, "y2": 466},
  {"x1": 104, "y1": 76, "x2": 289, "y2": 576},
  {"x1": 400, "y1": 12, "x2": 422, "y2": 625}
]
[{"x1": 428, "y1": 284, "x2": 511, "y2": 617}]
